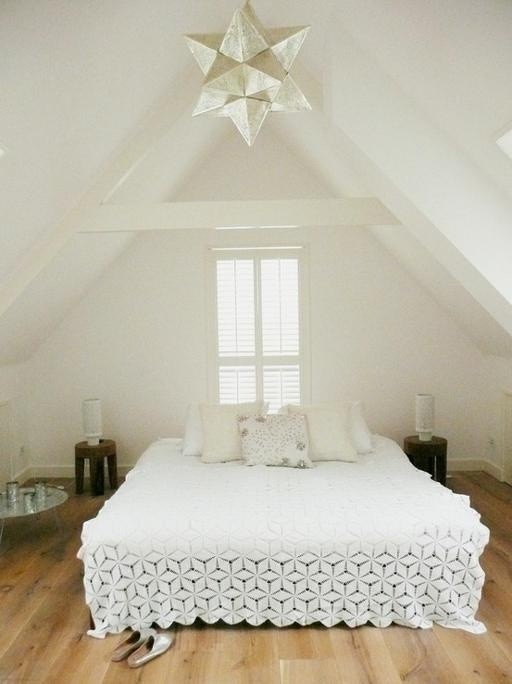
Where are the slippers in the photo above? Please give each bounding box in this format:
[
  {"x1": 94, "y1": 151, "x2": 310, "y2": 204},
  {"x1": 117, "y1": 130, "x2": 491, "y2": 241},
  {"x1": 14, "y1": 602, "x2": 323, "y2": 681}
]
[{"x1": 110, "y1": 628, "x2": 172, "y2": 668}]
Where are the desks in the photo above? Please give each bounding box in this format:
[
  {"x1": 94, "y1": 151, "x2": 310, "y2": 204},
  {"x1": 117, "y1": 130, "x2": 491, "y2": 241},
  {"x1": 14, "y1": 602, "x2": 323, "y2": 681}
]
[
  {"x1": 75, "y1": 439, "x2": 118, "y2": 496},
  {"x1": 405, "y1": 435, "x2": 447, "y2": 487}
]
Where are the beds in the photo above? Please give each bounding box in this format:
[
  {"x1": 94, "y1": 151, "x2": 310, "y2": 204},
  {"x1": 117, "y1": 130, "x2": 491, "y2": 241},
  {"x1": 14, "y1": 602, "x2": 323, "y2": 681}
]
[{"x1": 76, "y1": 435, "x2": 490, "y2": 639}]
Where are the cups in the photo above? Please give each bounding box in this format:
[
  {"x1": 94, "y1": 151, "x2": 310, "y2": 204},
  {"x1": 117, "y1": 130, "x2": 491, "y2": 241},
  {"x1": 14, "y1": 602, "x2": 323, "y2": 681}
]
[
  {"x1": 34, "y1": 480, "x2": 47, "y2": 502},
  {"x1": 4, "y1": 481, "x2": 20, "y2": 503}
]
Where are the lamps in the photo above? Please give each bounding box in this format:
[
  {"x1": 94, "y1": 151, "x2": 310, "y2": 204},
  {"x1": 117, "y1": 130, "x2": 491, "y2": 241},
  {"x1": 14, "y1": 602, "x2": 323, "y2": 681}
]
[
  {"x1": 416, "y1": 394, "x2": 435, "y2": 442},
  {"x1": 184, "y1": 0, "x2": 311, "y2": 147},
  {"x1": 82, "y1": 399, "x2": 102, "y2": 446}
]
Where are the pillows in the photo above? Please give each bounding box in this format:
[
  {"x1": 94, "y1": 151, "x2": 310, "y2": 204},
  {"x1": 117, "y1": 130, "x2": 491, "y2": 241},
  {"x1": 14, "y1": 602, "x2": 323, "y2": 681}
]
[{"x1": 181, "y1": 400, "x2": 376, "y2": 469}]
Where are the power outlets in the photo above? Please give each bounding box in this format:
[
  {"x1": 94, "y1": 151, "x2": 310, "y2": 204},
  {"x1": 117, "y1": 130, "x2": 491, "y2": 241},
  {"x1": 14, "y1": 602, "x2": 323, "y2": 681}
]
[{"x1": 20, "y1": 445, "x2": 25, "y2": 455}]
